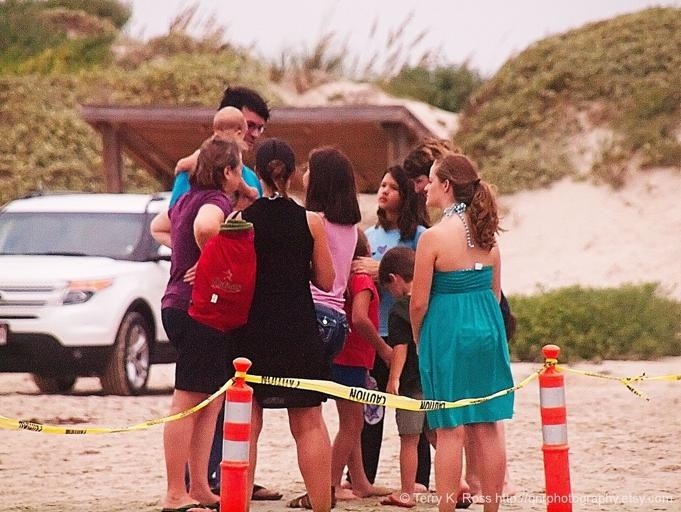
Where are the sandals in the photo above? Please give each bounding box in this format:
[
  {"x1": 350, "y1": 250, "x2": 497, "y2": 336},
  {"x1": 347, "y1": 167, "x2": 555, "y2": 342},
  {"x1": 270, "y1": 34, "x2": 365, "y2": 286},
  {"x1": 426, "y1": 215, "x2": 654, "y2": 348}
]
[{"x1": 286, "y1": 484, "x2": 336, "y2": 508}]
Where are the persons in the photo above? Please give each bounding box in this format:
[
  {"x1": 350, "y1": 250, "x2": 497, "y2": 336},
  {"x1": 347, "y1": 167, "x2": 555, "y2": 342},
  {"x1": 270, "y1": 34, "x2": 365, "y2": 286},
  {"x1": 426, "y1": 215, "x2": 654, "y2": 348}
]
[{"x1": 150, "y1": 86, "x2": 516, "y2": 512}]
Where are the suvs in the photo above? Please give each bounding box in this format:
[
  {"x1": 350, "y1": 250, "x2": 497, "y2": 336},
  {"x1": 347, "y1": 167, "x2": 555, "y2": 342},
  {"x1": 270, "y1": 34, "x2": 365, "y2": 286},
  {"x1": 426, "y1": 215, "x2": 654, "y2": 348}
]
[{"x1": 0, "y1": 189, "x2": 179, "y2": 396}]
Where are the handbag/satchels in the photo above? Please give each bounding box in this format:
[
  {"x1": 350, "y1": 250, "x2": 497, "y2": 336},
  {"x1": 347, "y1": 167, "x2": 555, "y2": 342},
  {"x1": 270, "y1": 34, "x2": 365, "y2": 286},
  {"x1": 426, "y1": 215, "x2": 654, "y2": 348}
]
[{"x1": 186, "y1": 221, "x2": 259, "y2": 332}]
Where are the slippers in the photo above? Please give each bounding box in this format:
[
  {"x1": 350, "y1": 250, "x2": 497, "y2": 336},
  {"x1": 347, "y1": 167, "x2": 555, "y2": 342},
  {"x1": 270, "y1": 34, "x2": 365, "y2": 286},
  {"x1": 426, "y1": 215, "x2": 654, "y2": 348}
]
[
  {"x1": 154, "y1": 485, "x2": 282, "y2": 512},
  {"x1": 380, "y1": 495, "x2": 416, "y2": 508},
  {"x1": 456, "y1": 496, "x2": 473, "y2": 508}
]
